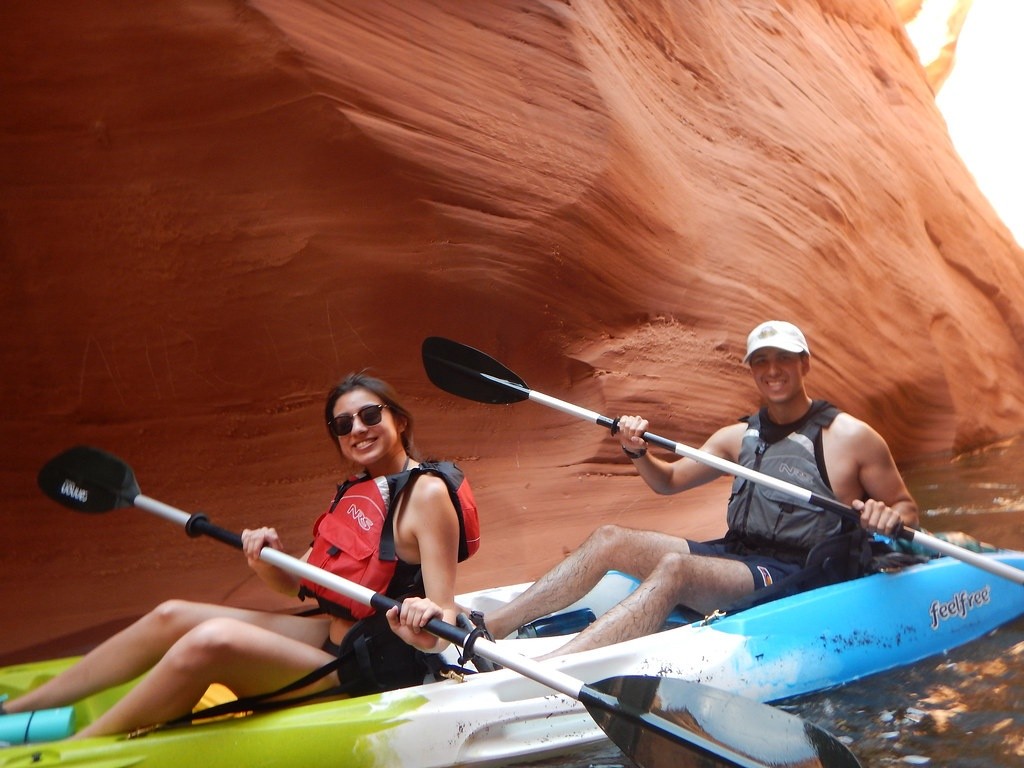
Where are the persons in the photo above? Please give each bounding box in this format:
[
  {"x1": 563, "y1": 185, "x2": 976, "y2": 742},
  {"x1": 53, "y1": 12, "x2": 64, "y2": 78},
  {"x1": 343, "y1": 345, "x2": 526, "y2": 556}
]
[
  {"x1": 453, "y1": 319, "x2": 921, "y2": 676},
  {"x1": 1, "y1": 376, "x2": 461, "y2": 743}
]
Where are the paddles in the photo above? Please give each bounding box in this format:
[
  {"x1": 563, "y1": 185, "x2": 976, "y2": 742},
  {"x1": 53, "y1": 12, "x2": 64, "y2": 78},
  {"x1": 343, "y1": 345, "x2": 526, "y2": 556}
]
[
  {"x1": 420, "y1": 335, "x2": 1024, "y2": 583},
  {"x1": 38, "y1": 443, "x2": 862, "y2": 768}
]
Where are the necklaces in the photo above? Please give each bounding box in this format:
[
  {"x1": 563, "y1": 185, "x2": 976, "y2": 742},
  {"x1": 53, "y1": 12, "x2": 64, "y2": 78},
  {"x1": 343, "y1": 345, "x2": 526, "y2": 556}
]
[{"x1": 402, "y1": 455, "x2": 410, "y2": 471}]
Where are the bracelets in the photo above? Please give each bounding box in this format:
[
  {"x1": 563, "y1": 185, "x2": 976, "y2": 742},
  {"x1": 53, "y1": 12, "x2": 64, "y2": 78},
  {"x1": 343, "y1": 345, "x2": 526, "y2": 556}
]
[{"x1": 620, "y1": 445, "x2": 647, "y2": 458}]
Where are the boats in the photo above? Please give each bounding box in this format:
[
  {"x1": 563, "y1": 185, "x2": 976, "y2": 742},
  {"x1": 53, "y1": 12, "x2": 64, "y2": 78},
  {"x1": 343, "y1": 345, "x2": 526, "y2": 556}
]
[{"x1": 0, "y1": 530, "x2": 1023, "y2": 767}]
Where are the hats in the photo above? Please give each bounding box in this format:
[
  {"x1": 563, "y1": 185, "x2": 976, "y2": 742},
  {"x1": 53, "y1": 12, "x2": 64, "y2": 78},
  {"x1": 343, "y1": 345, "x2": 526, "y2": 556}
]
[{"x1": 743, "y1": 320, "x2": 809, "y2": 363}]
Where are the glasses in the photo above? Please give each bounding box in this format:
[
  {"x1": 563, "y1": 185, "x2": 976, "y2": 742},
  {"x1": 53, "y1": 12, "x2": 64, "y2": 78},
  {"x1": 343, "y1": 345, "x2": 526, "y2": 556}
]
[{"x1": 328, "y1": 405, "x2": 389, "y2": 436}]
